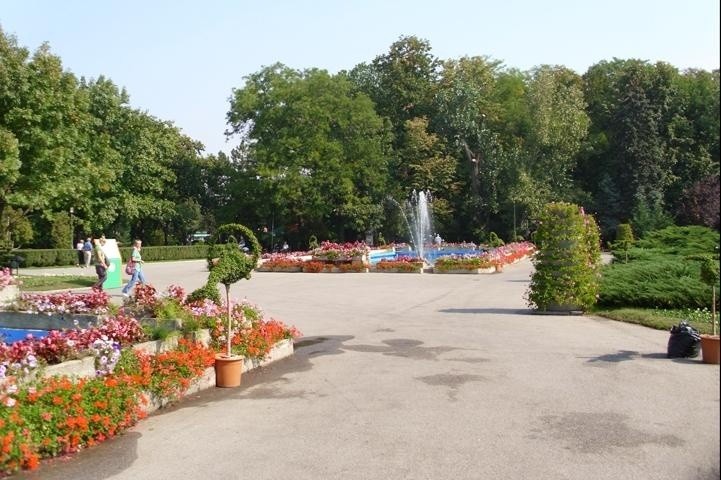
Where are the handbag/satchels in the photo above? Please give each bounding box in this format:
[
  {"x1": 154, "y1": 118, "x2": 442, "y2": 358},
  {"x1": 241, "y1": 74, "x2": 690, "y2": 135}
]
[{"x1": 125, "y1": 257, "x2": 135, "y2": 275}]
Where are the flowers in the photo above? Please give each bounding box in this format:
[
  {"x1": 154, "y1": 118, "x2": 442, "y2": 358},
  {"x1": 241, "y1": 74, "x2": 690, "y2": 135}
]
[
  {"x1": 435, "y1": 240, "x2": 537, "y2": 272},
  {"x1": 263, "y1": 239, "x2": 429, "y2": 273}
]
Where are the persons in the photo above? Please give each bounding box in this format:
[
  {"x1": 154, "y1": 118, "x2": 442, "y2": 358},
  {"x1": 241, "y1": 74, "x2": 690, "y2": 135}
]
[
  {"x1": 273, "y1": 241, "x2": 289, "y2": 253},
  {"x1": 76, "y1": 237, "x2": 93, "y2": 267},
  {"x1": 434, "y1": 234, "x2": 442, "y2": 244},
  {"x1": 92, "y1": 239, "x2": 108, "y2": 294},
  {"x1": 122, "y1": 239, "x2": 147, "y2": 297}
]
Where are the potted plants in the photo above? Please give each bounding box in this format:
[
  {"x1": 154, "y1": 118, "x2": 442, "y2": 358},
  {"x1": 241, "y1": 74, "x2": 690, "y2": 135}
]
[
  {"x1": 182, "y1": 224, "x2": 261, "y2": 389},
  {"x1": 697, "y1": 255, "x2": 721, "y2": 365},
  {"x1": 522, "y1": 200, "x2": 602, "y2": 313}
]
